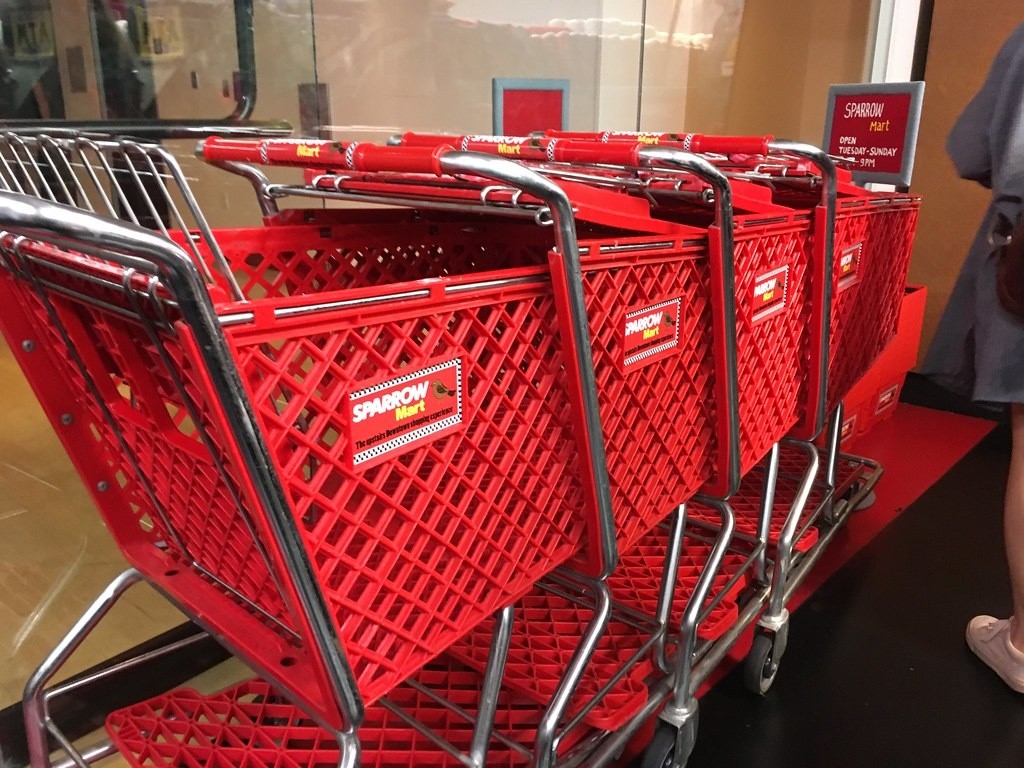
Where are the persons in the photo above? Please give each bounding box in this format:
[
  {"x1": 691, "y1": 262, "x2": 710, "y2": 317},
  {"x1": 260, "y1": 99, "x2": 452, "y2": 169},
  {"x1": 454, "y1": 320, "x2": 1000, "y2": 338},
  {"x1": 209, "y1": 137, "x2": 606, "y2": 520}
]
[
  {"x1": 0, "y1": 40, "x2": 51, "y2": 196},
  {"x1": 923, "y1": 22, "x2": 1024, "y2": 692}
]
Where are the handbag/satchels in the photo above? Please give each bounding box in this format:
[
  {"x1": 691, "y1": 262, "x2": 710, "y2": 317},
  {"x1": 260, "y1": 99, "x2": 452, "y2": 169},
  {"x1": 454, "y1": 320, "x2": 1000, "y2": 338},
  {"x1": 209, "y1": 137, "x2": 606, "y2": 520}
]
[{"x1": 995, "y1": 208, "x2": 1024, "y2": 317}]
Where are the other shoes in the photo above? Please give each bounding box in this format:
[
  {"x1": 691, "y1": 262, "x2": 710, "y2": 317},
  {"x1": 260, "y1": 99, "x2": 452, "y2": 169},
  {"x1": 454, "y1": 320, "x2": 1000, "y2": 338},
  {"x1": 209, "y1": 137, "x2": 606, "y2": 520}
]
[{"x1": 966, "y1": 615, "x2": 1024, "y2": 693}]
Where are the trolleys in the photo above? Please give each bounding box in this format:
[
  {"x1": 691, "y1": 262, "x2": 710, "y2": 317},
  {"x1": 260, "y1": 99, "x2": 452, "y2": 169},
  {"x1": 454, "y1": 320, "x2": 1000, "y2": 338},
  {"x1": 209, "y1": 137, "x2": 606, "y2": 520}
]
[{"x1": 1, "y1": 76, "x2": 929, "y2": 766}]
